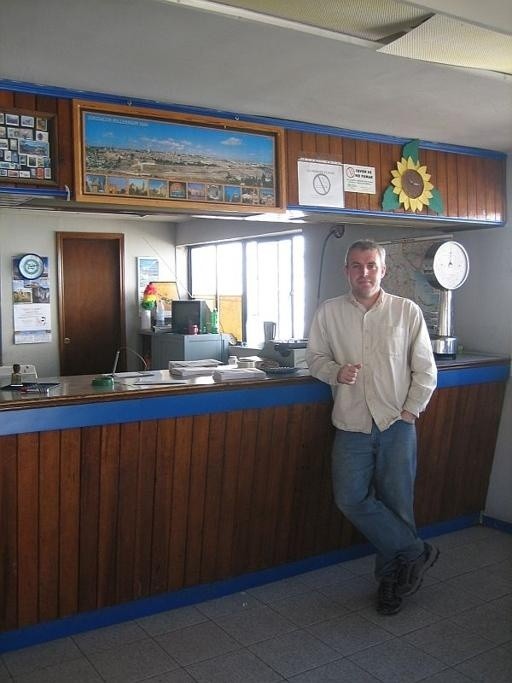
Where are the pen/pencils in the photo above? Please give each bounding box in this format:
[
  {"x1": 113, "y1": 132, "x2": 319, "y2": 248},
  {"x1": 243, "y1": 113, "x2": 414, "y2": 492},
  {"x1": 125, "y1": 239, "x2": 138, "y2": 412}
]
[{"x1": 112, "y1": 350, "x2": 120, "y2": 376}]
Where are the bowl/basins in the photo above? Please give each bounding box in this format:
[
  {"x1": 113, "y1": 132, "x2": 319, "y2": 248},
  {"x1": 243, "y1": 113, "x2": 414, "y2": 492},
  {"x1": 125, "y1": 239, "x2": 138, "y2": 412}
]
[{"x1": 237, "y1": 361, "x2": 255, "y2": 367}]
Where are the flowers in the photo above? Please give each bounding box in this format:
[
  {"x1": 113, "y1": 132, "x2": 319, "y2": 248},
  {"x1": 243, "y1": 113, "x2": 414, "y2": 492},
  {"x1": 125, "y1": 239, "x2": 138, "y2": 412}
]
[
  {"x1": 141, "y1": 284, "x2": 156, "y2": 309},
  {"x1": 377, "y1": 137, "x2": 441, "y2": 215}
]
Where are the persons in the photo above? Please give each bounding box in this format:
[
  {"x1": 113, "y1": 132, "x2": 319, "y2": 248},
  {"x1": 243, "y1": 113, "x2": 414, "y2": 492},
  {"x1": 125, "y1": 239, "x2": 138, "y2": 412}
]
[{"x1": 304, "y1": 239, "x2": 438, "y2": 615}]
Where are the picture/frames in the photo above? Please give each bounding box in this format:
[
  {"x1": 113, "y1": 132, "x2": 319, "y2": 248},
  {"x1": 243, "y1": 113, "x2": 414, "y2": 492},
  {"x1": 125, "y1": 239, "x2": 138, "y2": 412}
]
[
  {"x1": 71, "y1": 94, "x2": 288, "y2": 212},
  {"x1": 1, "y1": 105, "x2": 62, "y2": 186},
  {"x1": 136, "y1": 254, "x2": 160, "y2": 300},
  {"x1": 150, "y1": 279, "x2": 181, "y2": 318}
]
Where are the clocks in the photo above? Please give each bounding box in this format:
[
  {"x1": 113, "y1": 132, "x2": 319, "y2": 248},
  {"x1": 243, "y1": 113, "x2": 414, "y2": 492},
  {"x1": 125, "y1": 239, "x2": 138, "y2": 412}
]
[
  {"x1": 17, "y1": 251, "x2": 45, "y2": 279},
  {"x1": 415, "y1": 240, "x2": 470, "y2": 360}
]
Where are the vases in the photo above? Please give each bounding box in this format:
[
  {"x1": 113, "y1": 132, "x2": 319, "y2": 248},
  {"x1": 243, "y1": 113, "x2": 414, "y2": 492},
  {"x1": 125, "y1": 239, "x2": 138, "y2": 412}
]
[{"x1": 140, "y1": 308, "x2": 152, "y2": 330}]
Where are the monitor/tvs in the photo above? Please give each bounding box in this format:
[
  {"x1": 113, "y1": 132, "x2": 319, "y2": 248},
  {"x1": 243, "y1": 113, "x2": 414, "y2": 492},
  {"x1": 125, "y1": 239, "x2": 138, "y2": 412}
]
[{"x1": 171, "y1": 300, "x2": 213, "y2": 334}]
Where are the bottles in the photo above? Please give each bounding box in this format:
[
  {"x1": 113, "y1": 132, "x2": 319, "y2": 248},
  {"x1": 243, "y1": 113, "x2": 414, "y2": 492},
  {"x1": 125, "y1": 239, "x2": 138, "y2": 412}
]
[
  {"x1": 11, "y1": 364, "x2": 23, "y2": 385},
  {"x1": 192, "y1": 323, "x2": 199, "y2": 334},
  {"x1": 157, "y1": 300, "x2": 165, "y2": 325},
  {"x1": 211, "y1": 308, "x2": 219, "y2": 333},
  {"x1": 228, "y1": 355, "x2": 238, "y2": 369}
]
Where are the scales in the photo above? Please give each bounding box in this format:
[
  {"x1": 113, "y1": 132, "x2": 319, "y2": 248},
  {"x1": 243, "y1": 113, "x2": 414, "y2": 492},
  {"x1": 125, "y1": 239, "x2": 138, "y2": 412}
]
[{"x1": 422, "y1": 240, "x2": 470, "y2": 361}]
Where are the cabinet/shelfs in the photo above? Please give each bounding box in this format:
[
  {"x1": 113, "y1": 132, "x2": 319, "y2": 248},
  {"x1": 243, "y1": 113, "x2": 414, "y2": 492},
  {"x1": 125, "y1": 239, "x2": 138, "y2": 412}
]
[{"x1": 150, "y1": 333, "x2": 225, "y2": 369}]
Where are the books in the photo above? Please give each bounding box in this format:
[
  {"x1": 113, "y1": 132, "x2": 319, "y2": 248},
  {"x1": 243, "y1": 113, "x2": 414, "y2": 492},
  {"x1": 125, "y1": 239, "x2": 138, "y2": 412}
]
[{"x1": 169, "y1": 358, "x2": 266, "y2": 383}]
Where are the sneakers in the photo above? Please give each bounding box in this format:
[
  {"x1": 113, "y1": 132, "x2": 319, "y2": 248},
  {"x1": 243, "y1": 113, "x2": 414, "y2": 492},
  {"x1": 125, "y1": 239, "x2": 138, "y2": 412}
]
[
  {"x1": 375, "y1": 578, "x2": 402, "y2": 615},
  {"x1": 395, "y1": 542, "x2": 440, "y2": 598}
]
